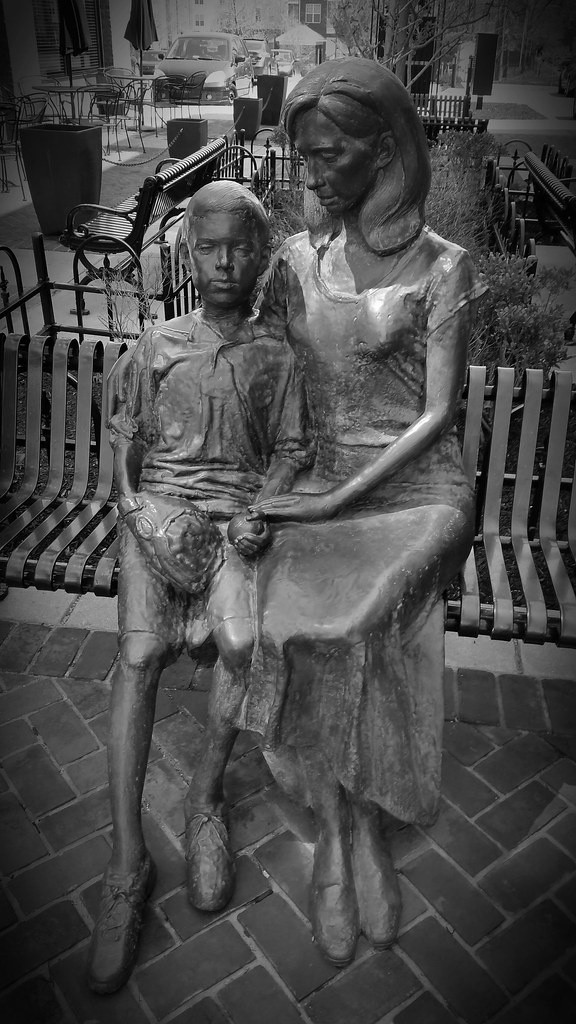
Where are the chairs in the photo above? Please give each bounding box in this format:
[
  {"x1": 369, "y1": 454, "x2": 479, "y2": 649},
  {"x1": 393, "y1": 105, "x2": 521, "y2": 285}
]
[{"x1": 0, "y1": 65, "x2": 208, "y2": 202}]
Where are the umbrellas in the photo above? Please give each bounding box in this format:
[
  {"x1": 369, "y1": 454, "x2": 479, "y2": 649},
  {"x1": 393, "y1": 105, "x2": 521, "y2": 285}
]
[{"x1": 56, "y1": 0, "x2": 158, "y2": 124}]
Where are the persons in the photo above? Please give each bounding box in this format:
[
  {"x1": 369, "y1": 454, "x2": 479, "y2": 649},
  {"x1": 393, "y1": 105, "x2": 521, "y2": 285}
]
[
  {"x1": 82, "y1": 180, "x2": 308, "y2": 1001},
  {"x1": 103, "y1": 57, "x2": 494, "y2": 968}
]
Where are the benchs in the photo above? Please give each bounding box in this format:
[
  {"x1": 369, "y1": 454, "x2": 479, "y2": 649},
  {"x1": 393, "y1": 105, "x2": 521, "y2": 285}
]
[
  {"x1": 0, "y1": 332, "x2": 576, "y2": 648},
  {"x1": 59, "y1": 135, "x2": 228, "y2": 319},
  {"x1": 524, "y1": 151, "x2": 576, "y2": 342}
]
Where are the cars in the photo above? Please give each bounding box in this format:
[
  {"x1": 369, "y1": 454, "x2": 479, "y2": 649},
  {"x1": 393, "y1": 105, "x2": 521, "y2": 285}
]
[
  {"x1": 149, "y1": 31, "x2": 256, "y2": 106},
  {"x1": 136, "y1": 37, "x2": 299, "y2": 86}
]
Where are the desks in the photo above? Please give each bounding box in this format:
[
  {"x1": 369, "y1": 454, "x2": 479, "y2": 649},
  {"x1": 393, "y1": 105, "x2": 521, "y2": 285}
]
[
  {"x1": 31, "y1": 85, "x2": 113, "y2": 156},
  {"x1": 114, "y1": 76, "x2": 170, "y2": 131}
]
